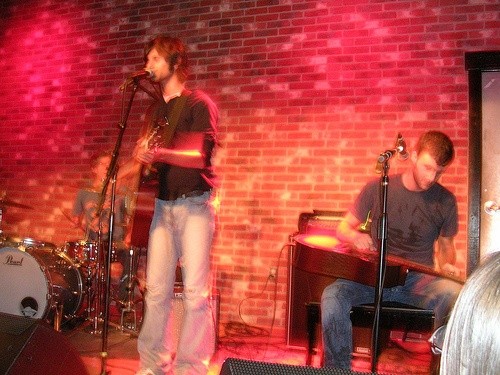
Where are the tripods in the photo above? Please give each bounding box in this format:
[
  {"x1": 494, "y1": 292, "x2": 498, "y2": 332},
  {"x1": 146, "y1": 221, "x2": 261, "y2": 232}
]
[{"x1": 62, "y1": 239, "x2": 138, "y2": 337}]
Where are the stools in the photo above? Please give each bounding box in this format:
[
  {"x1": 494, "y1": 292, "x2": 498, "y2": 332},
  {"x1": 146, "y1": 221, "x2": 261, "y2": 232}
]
[{"x1": 306, "y1": 300, "x2": 434, "y2": 370}]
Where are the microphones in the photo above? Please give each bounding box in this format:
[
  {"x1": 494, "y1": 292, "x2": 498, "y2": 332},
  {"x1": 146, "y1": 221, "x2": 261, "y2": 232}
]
[
  {"x1": 397, "y1": 134, "x2": 409, "y2": 160},
  {"x1": 124, "y1": 68, "x2": 153, "y2": 78}
]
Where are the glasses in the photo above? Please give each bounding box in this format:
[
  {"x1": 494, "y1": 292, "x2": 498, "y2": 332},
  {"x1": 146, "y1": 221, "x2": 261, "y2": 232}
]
[{"x1": 427, "y1": 325, "x2": 446, "y2": 355}]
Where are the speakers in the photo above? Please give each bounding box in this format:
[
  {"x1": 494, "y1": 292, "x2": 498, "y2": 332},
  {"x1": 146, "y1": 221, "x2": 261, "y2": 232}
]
[
  {"x1": 218, "y1": 357, "x2": 367, "y2": 375},
  {"x1": 0, "y1": 311, "x2": 91, "y2": 375}
]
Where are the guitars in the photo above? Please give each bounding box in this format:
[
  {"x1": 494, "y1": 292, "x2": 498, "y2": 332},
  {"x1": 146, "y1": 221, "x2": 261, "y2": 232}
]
[
  {"x1": 121, "y1": 114, "x2": 171, "y2": 248},
  {"x1": 292, "y1": 230, "x2": 468, "y2": 289}
]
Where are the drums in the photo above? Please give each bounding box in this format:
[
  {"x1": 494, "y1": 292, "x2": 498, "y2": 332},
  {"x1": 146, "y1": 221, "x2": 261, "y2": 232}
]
[
  {"x1": 6, "y1": 235, "x2": 56, "y2": 249},
  {"x1": 0, "y1": 242, "x2": 86, "y2": 324},
  {"x1": 65, "y1": 239, "x2": 116, "y2": 268}
]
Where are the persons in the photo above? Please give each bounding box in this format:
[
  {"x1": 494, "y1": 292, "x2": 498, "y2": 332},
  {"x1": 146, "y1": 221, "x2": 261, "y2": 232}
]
[
  {"x1": 133, "y1": 34, "x2": 221, "y2": 375},
  {"x1": 321, "y1": 130, "x2": 463, "y2": 370},
  {"x1": 440, "y1": 251, "x2": 500, "y2": 375}
]
